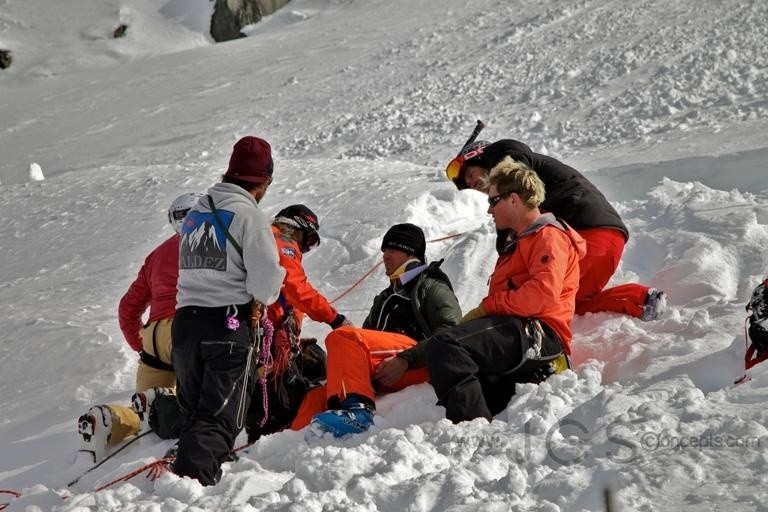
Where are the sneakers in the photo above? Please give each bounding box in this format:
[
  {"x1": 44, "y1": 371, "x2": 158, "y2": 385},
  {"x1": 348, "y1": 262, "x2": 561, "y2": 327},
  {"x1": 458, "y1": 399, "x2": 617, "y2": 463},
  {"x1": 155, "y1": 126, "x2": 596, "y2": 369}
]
[
  {"x1": 640, "y1": 286, "x2": 668, "y2": 322},
  {"x1": 75, "y1": 403, "x2": 113, "y2": 473},
  {"x1": 307, "y1": 403, "x2": 375, "y2": 437},
  {"x1": 130, "y1": 386, "x2": 176, "y2": 434}
]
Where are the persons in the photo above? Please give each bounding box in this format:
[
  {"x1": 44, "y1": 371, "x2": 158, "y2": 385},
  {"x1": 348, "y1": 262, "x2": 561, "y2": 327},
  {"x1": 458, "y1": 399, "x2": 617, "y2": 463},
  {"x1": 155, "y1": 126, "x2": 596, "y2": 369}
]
[
  {"x1": 293, "y1": 222, "x2": 462, "y2": 437},
  {"x1": 243, "y1": 203, "x2": 352, "y2": 444},
  {"x1": 76, "y1": 192, "x2": 207, "y2": 465},
  {"x1": 151, "y1": 134, "x2": 287, "y2": 487},
  {"x1": 445, "y1": 140, "x2": 669, "y2": 322},
  {"x1": 425, "y1": 160, "x2": 588, "y2": 423}
]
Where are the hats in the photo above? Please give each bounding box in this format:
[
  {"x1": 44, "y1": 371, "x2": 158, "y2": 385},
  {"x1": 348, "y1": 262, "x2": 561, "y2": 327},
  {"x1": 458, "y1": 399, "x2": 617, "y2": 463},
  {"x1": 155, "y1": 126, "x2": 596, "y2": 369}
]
[
  {"x1": 222, "y1": 135, "x2": 275, "y2": 188},
  {"x1": 446, "y1": 139, "x2": 493, "y2": 186},
  {"x1": 167, "y1": 190, "x2": 201, "y2": 235},
  {"x1": 271, "y1": 205, "x2": 321, "y2": 254},
  {"x1": 380, "y1": 221, "x2": 427, "y2": 264}
]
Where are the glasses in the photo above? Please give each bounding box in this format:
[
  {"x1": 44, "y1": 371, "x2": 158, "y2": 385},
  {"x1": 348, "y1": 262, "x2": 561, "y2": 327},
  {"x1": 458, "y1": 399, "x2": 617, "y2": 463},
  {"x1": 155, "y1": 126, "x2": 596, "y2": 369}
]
[
  {"x1": 446, "y1": 157, "x2": 465, "y2": 183},
  {"x1": 486, "y1": 182, "x2": 524, "y2": 209},
  {"x1": 304, "y1": 229, "x2": 320, "y2": 252}
]
